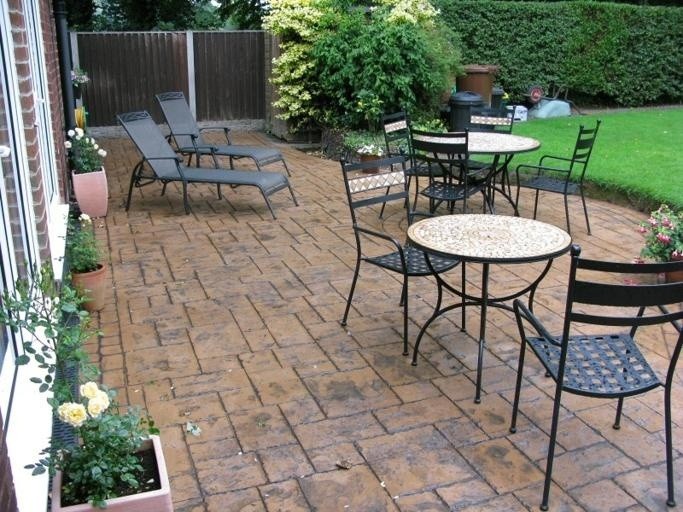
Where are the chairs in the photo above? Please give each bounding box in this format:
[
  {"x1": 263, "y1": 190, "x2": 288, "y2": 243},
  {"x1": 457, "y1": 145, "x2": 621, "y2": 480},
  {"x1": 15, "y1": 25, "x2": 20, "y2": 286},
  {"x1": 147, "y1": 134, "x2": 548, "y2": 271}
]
[
  {"x1": 156, "y1": 91, "x2": 292, "y2": 188},
  {"x1": 510, "y1": 244, "x2": 683, "y2": 512},
  {"x1": 340, "y1": 146, "x2": 465, "y2": 355},
  {"x1": 378, "y1": 100, "x2": 601, "y2": 236},
  {"x1": 116, "y1": 110, "x2": 299, "y2": 220}
]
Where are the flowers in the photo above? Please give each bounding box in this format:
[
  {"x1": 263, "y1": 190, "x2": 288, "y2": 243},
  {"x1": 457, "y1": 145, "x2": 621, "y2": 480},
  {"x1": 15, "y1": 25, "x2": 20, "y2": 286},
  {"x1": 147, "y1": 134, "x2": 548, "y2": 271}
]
[
  {"x1": 58, "y1": 213, "x2": 98, "y2": 271},
  {"x1": 64, "y1": 128, "x2": 107, "y2": 173},
  {"x1": 70, "y1": 67, "x2": 90, "y2": 87},
  {"x1": 356, "y1": 144, "x2": 383, "y2": 157},
  {"x1": 637, "y1": 204, "x2": 683, "y2": 260},
  {"x1": 0, "y1": 259, "x2": 159, "y2": 509}
]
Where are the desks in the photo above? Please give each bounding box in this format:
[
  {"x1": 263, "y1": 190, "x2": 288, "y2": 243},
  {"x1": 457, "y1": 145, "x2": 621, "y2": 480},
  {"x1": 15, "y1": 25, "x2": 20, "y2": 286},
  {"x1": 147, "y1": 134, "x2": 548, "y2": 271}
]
[{"x1": 406, "y1": 214, "x2": 572, "y2": 404}]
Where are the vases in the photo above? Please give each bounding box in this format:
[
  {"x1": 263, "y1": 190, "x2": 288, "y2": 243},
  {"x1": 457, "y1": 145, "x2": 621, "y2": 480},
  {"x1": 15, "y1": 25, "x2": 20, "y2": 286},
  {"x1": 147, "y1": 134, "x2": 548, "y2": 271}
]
[
  {"x1": 360, "y1": 156, "x2": 379, "y2": 173},
  {"x1": 72, "y1": 166, "x2": 108, "y2": 218},
  {"x1": 51, "y1": 434, "x2": 173, "y2": 512},
  {"x1": 69, "y1": 264, "x2": 106, "y2": 311},
  {"x1": 72, "y1": 83, "x2": 81, "y2": 99},
  {"x1": 665, "y1": 260, "x2": 683, "y2": 284}
]
